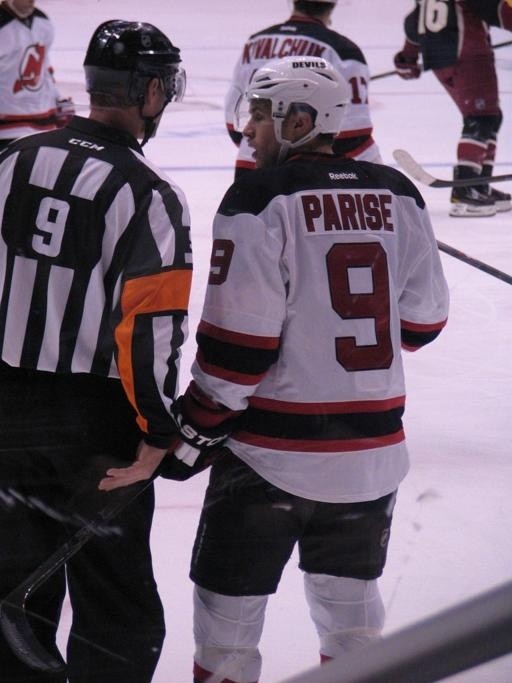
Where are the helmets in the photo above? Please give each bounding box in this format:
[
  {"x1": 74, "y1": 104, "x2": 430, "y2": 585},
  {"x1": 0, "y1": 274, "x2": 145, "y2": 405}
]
[
  {"x1": 83, "y1": 19, "x2": 182, "y2": 107},
  {"x1": 247, "y1": 55, "x2": 351, "y2": 134}
]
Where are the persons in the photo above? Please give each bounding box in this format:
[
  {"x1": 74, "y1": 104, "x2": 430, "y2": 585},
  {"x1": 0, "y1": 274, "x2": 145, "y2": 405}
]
[
  {"x1": 0, "y1": 0, "x2": 76, "y2": 154},
  {"x1": 0, "y1": 20, "x2": 193, "y2": 683},
  {"x1": 224, "y1": 0, "x2": 383, "y2": 184},
  {"x1": 394, "y1": 0, "x2": 512, "y2": 217},
  {"x1": 161, "y1": 55, "x2": 451, "y2": 683}
]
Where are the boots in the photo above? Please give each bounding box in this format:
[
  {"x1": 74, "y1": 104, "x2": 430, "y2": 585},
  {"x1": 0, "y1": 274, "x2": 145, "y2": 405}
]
[{"x1": 450, "y1": 161, "x2": 511, "y2": 207}]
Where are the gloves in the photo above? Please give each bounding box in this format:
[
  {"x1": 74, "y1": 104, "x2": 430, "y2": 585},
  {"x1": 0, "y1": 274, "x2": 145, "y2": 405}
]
[
  {"x1": 159, "y1": 380, "x2": 239, "y2": 481},
  {"x1": 393, "y1": 40, "x2": 420, "y2": 80}
]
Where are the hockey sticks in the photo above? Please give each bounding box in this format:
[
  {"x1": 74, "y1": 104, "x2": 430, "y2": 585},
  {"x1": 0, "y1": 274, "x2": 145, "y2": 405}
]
[
  {"x1": 1, "y1": 474, "x2": 161, "y2": 675},
  {"x1": 393, "y1": 149, "x2": 512, "y2": 187}
]
